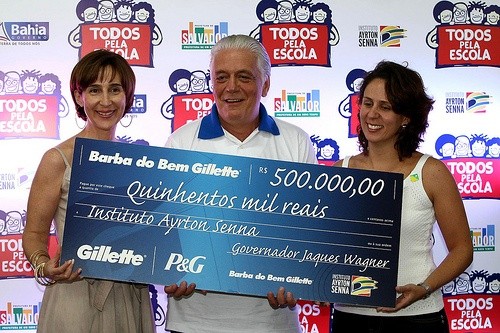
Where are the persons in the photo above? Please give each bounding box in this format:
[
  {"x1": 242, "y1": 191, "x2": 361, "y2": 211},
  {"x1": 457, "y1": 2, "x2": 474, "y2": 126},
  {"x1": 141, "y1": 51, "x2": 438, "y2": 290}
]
[
  {"x1": 21, "y1": 49, "x2": 159, "y2": 333},
  {"x1": 160, "y1": 33, "x2": 321, "y2": 333},
  {"x1": 328, "y1": 58, "x2": 475, "y2": 333}
]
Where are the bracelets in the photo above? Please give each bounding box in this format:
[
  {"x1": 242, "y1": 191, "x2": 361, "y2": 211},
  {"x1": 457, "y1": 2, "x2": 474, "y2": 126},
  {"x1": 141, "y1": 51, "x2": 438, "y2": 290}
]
[
  {"x1": 29, "y1": 249, "x2": 56, "y2": 287},
  {"x1": 417, "y1": 283, "x2": 432, "y2": 299}
]
